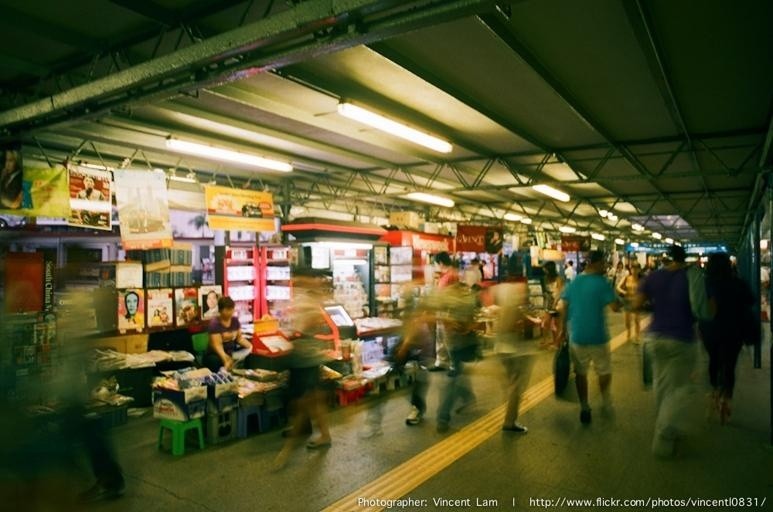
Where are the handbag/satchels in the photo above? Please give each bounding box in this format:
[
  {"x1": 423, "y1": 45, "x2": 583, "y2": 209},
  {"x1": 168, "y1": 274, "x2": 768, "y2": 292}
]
[{"x1": 554, "y1": 340, "x2": 569, "y2": 396}]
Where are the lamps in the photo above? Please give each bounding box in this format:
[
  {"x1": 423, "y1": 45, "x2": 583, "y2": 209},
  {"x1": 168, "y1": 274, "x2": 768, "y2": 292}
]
[
  {"x1": 337, "y1": 96, "x2": 454, "y2": 155},
  {"x1": 165, "y1": 134, "x2": 293, "y2": 174}
]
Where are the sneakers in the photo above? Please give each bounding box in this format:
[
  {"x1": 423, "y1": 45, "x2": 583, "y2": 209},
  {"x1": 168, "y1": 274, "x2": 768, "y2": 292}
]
[
  {"x1": 79, "y1": 482, "x2": 126, "y2": 503},
  {"x1": 502, "y1": 423, "x2": 528, "y2": 433},
  {"x1": 406, "y1": 405, "x2": 426, "y2": 425},
  {"x1": 580, "y1": 404, "x2": 591, "y2": 422}
]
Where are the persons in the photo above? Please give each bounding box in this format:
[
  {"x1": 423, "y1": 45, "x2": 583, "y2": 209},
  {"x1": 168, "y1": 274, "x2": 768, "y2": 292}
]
[
  {"x1": 207, "y1": 295, "x2": 253, "y2": 373},
  {"x1": 68, "y1": 403, "x2": 127, "y2": 503},
  {"x1": 151, "y1": 309, "x2": 161, "y2": 325},
  {"x1": 270, "y1": 246, "x2": 757, "y2": 474},
  {"x1": 76, "y1": 175, "x2": 108, "y2": 227},
  {"x1": 124, "y1": 291, "x2": 139, "y2": 324},
  {"x1": 159, "y1": 306, "x2": 169, "y2": 323},
  {"x1": 204, "y1": 290, "x2": 219, "y2": 318},
  {"x1": 0, "y1": 149, "x2": 23, "y2": 209}
]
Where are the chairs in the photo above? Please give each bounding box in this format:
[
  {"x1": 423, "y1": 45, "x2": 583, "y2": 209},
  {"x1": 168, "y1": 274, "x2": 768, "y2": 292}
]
[{"x1": 190, "y1": 330, "x2": 210, "y2": 368}]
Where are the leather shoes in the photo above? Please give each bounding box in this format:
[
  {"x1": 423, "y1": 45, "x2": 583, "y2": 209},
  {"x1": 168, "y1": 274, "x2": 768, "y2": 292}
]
[
  {"x1": 448, "y1": 370, "x2": 456, "y2": 376},
  {"x1": 428, "y1": 365, "x2": 445, "y2": 372},
  {"x1": 308, "y1": 438, "x2": 331, "y2": 448}
]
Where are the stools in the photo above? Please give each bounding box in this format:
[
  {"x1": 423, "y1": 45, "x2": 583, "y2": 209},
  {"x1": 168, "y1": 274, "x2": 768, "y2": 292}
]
[
  {"x1": 156, "y1": 418, "x2": 205, "y2": 457},
  {"x1": 333, "y1": 367, "x2": 416, "y2": 407},
  {"x1": 236, "y1": 405, "x2": 262, "y2": 439}
]
